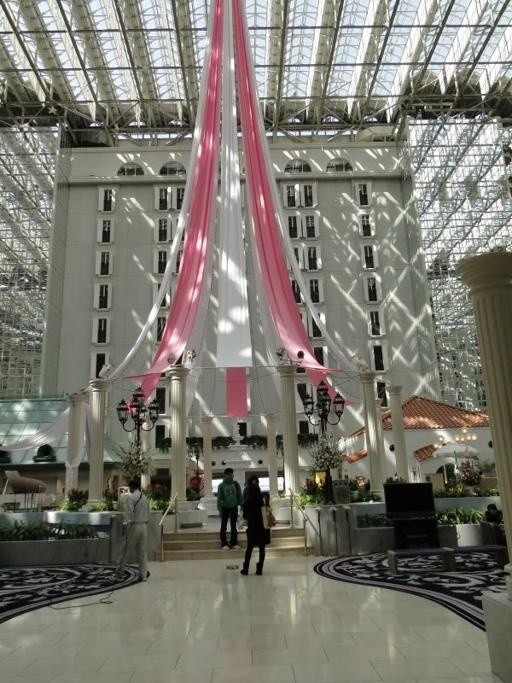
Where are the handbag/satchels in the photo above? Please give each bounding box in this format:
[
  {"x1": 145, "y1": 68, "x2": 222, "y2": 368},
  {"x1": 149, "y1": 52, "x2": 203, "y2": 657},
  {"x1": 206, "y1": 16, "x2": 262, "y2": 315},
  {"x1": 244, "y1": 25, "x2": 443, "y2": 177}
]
[{"x1": 261, "y1": 504, "x2": 278, "y2": 529}]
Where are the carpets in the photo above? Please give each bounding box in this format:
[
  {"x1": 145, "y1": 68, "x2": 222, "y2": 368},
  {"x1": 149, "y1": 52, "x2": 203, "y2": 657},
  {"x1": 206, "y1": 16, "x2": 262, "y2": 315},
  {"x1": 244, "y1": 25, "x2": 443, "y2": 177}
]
[
  {"x1": 313, "y1": 544, "x2": 510, "y2": 631},
  {"x1": 0, "y1": 565, "x2": 150, "y2": 623}
]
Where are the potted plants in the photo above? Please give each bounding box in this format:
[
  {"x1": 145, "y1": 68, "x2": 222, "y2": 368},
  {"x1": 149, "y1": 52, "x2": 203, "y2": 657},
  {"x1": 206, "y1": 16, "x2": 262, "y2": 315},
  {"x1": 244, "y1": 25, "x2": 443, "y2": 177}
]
[
  {"x1": 348, "y1": 478, "x2": 360, "y2": 502},
  {"x1": 101, "y1": 488, "x2": 119, "y2": 510},
  {"x1": 297, "y1": 482, "x2": 327, "y2": 510}
]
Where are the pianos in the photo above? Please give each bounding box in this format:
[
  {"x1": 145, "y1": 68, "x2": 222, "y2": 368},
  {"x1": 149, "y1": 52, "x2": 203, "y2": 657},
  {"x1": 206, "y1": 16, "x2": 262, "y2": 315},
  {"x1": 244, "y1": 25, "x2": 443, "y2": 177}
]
[{"x1": 0, "y1": 469, "x2": 47, "y2": 508}]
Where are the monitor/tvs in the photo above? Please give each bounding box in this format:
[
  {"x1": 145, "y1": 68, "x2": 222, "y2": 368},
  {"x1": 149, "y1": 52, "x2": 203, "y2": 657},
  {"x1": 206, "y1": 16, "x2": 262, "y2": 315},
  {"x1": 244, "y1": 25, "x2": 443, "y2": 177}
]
[{"x1": 383, "y1": 482, "x2": 435, "y2": 519}]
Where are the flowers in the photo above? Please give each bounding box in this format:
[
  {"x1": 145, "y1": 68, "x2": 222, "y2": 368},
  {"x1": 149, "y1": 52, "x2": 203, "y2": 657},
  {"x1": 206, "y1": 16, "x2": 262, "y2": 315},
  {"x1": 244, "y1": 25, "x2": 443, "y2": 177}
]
[
  {"x1": 64, "y1": 487, "x2": 90, "y2": 511},
  {"x1": 116, "y1": 440, "x2": 154, "y2": 473},
  {"x1": 453, "y1": 459, "x2": 480, "y2": 485},
  {"x1": 147, "y1": 484, "x2": 175, "y2": 501},
  {"x1": 190, "y1": 475, "x2": 205, "y2": 494},
  {"x1": 308, "y1": 432, "x2": 346, "y2": 470}
]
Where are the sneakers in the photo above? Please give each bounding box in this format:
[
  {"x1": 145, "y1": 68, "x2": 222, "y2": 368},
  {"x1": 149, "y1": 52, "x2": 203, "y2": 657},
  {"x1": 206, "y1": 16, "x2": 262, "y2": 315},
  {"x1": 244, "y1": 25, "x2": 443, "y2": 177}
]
[
  {"x1": 231, "y1": 544, "x2": 242, "y2": 549},
  {"x1": 221, "y1": 544, "x2": 230, "y2": 549},
  {"x1": 108, "y1": 574, "x2": 124, "y2": 582}
]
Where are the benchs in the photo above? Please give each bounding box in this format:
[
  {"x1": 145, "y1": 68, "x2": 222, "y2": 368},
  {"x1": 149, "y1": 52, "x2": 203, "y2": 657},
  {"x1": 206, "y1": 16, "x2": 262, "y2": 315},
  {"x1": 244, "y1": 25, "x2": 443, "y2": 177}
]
[
  {"x1": 4, "y1": 502, "x2": 21, "y2": 512},
  {"x1": 387, "y1": 545, "x2": 509, "y2": 575}
]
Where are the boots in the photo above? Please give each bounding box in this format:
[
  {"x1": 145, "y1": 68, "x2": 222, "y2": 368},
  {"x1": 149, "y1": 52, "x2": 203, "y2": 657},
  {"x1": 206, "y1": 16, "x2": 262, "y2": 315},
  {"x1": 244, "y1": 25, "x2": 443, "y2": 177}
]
[
  {"x1": 240, "y1": 562, "x2": 249, "y2": 575},
  {"x1": 256, "y1": 562, "x2": 263, "y2": 575}
]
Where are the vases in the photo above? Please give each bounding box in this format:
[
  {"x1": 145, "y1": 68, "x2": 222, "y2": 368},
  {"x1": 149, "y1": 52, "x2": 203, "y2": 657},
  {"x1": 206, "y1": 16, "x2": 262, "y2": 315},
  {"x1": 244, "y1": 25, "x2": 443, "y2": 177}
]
[
  {"x1": 323, "y1": 467, "x2": 337, "y2": 505},
  {"x1": 463, "y1": 485, "x2": 478, "y2": 496},
  {"x1": 190, "y1": 489, "x2": 201, "y2": 500},
  {"x1": 150, "y1": 498, "x2": 164, "y2": 510}
]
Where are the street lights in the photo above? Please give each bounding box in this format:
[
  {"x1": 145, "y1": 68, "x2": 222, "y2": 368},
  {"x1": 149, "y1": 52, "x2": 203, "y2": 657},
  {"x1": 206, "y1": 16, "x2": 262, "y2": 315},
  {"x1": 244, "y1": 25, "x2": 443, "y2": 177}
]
[
  {"x1": 116, "y1": 384, "x2": 160, "y2": 490},
  {"x1": 302, "y1": 379, "x2": 346, "y2": 503}
]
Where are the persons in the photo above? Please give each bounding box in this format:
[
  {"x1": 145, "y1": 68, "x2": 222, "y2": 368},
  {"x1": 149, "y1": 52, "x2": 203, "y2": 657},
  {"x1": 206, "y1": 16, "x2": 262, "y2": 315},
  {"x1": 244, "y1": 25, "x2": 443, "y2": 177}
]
[
  {"x1": 242, "y1": 474, "x2": 261, "y2": 501},
  {"x1": 479, "y1": 504, "x2": 503, "y2": 544},
  {"x1": 239, "y1": 484, "x2": 271, "y2": 576},
  {"x1": 216, "y1": 467, "x2": 244, "y2": 551},
  {"x1": 114, "y1": 479, "x2": 152, "y2": 582}
]
